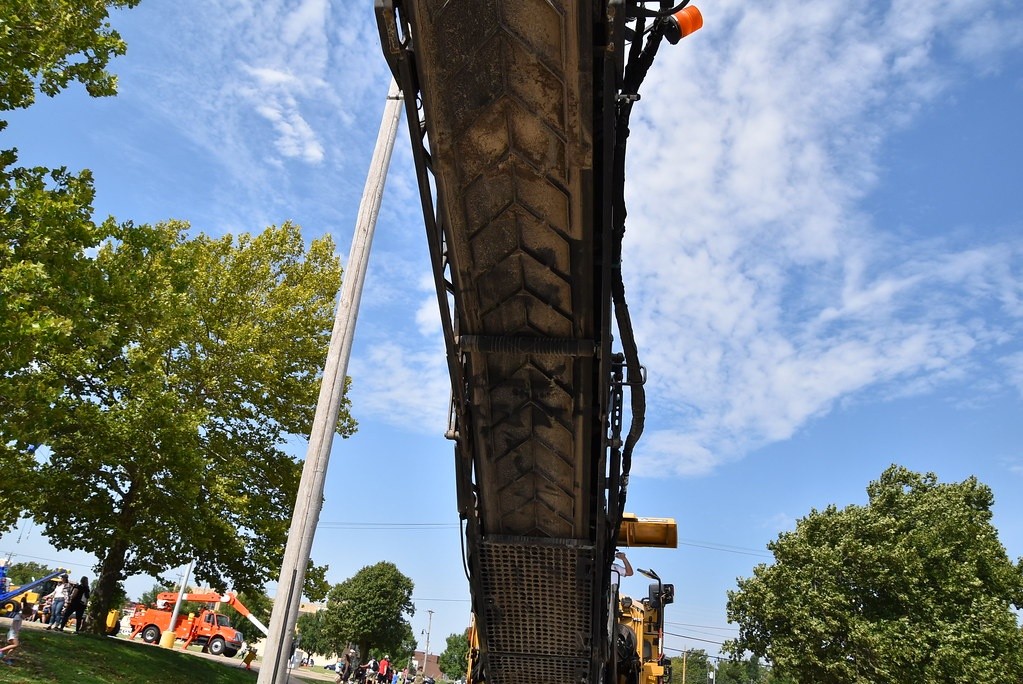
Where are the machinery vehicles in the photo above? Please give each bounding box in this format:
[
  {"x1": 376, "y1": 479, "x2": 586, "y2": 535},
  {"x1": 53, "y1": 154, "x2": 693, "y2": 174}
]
[
  {"x1": 463, "y1": 515, "x2": 678, "y2": 684},
  {"x1": 0, "y1": 568, "x2": 120, "y2": 637},
  {"x1": 128, "y1": 591, "x2": 268, "y2": 655}
]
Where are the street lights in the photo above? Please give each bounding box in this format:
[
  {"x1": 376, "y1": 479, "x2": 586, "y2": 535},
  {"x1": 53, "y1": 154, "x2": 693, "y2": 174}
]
[{"x1": 420, "y1": 608, "x2": 435, "y2": 674}]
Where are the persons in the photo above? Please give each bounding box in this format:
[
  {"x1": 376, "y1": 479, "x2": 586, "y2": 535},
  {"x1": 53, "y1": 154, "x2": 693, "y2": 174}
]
[
  {"x1": 0, "y1": 607, "x2": 33, "y2": 666},
  {"x1": 336, "y1": 653, "x2": 425, "y2": 684},
  {"x1": 20, "y1": 573, "x2": 90, "y2": 633}
]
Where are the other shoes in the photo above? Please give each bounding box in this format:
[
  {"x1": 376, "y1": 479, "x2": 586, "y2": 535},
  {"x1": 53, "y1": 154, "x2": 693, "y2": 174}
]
[
  {"x1": 53, "y1": 626, "x2": 58, "y2": 631},
  {"x1": 46, "y1": 626, "x2": 51, "y2": 630},
  {"x1": 1, "y1": 657, "x2": 13, "y2": 664},
  {"x1": 0, "y1": 648, "x2": 3, "y2": 657}
]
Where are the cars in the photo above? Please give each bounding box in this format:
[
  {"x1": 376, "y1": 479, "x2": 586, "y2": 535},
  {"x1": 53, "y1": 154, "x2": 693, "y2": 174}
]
[{"x1": 324, "y1": 664, "x2": 352, "y2": 672}]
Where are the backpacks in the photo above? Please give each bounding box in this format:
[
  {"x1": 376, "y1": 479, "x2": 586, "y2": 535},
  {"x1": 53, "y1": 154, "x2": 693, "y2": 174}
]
[
  {"x1": 371, "y1": 659, "x2": 378, "y2": 671},
  {"x1": 80, "y1": 585, "x2": 90, "y2": 606}
]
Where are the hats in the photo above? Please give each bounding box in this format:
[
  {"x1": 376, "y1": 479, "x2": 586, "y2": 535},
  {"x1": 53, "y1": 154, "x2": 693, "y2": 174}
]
[
  {"x1": 385, "y1": 655, "x2": 389, "y2": 659},
  {"x1": 350, "y1": 649, "x2": 355, "y2": 653},
  {"x1": 60, "y1": 574, "x2": 68, "y2": 578}
]
